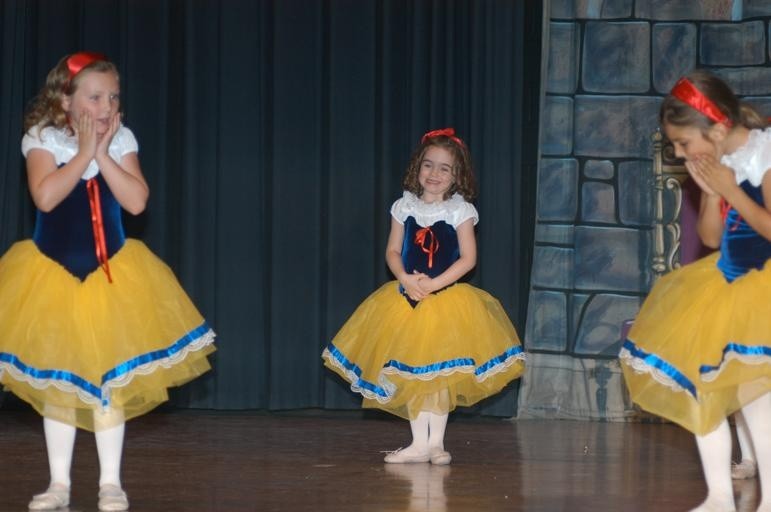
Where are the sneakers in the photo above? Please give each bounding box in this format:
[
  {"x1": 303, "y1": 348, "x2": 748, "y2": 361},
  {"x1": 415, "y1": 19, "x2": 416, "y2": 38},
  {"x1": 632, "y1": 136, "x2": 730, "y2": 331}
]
[
  {"x1": 430, "y1": 451, "x2": 451, "y2": 464},
  {"x1": 28, "y1": 482, "x2": 70, "y2": 511},
  {"x1": 98, "y1": 483, "x2": 129, "y2": 512},
  {"x1": 731, "y1": 459, "x2": 756, "y2": 479},
  {"x1": 380, "y1": 447, "x2": 430, "y2": 464}
]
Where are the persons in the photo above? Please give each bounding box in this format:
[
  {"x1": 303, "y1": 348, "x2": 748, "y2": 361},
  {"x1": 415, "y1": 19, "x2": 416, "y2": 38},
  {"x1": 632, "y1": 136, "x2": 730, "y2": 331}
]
[
  {"x1": 727, "y1": 97, "x2": 764, "y2": 478},
  {"x1": 322, "y1": 126, "x2": 527, "y2": 468},
  {"x1": 618, "y1": 74, "x2": 769, "y2": 511},
  {"x1": 0, "y1": 53, "x2": 221, "y2": 511}
]
